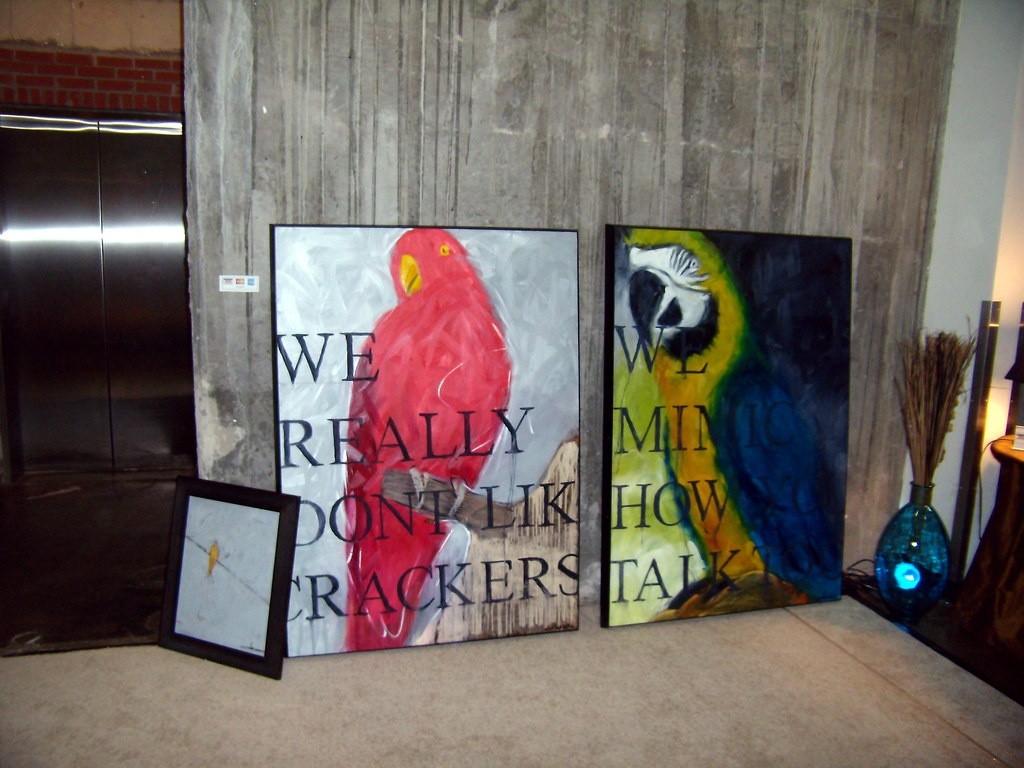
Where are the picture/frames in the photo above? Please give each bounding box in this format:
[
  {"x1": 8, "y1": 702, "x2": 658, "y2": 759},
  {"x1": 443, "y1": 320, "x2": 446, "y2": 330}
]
[{"x1": 156, "y1": 474, "x2": 301, "y2": 681}]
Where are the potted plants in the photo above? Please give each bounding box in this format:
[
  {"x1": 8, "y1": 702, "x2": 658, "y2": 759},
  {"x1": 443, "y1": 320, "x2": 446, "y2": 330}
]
[{"x1": 878, "y1": 314, "x2": 983, "y2": 622}]
